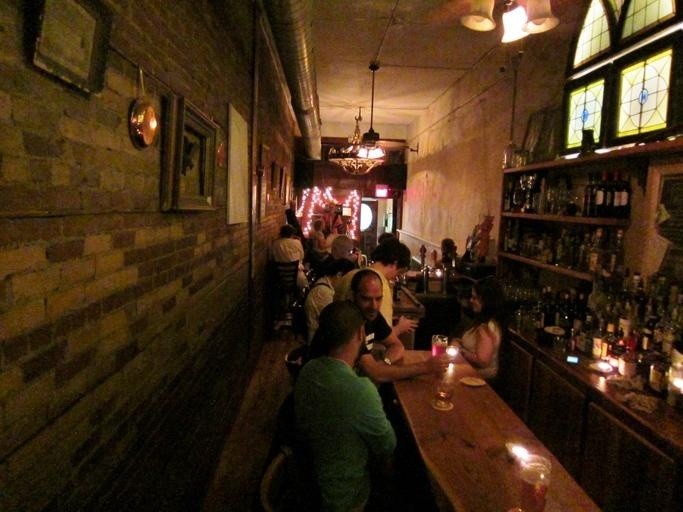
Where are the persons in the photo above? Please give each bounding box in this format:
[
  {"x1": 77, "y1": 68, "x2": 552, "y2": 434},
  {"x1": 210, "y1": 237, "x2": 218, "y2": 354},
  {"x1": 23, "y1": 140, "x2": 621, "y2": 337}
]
[
  {"x1": 440, "y1": 237, "x2": 458, "y2": 266},
  {"x1": 351, "y1": 269, "x2": 454, "y2": 388},
  {"x1": 292, "y1": 300, "x2": 441, "y2": 511},
  {"x1": 449, "y1": 278, "x2": 507, "y2": 381},
  {"x1": 266, "y1": 217, "x2": 422, "y2": 349}
]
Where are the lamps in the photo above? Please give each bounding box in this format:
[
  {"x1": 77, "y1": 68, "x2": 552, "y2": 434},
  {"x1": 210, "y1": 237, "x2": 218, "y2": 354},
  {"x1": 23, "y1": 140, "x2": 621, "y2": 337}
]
[
  {"x1": 460, "y1": 0, "x2": 557, "y2": 44},
  {"x1": 324, "y1": 108, "x2": 384, "y2": 174},
  {"x1": 347, "y1": 63, "x2": 387, "y2": 159}
]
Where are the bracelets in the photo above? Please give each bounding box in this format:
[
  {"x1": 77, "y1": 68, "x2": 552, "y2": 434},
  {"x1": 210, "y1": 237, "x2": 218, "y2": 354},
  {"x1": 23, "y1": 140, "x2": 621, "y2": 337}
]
[{"x1": 383, "y1": 356, "x2": 392, "y2": 366}]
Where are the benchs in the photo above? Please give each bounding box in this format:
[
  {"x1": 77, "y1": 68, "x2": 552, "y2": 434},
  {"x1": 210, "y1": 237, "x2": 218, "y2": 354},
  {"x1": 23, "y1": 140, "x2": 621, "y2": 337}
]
[{"x1": 204, "y1": 333, "x2": 299, "y2": 512}]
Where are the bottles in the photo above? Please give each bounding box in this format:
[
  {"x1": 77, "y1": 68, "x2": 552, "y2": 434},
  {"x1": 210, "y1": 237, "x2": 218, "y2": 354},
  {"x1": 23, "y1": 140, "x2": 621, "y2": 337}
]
[
  {"x1": 502, "y1": 274, "x2": 682, "y2": 409},
  {"x1": 502, "y1": 174, "x2": 630, "y2": 218},
  {"x1": 513, "y1": 150, "x2": 530, "y2": 169},
  {"x1": 502, "y1": 139, "x2": 520, "y2": 169},
  {"x1": 500, "y1": 224, "x2": 626, "y2": 276},
  {"x1": 392, "y1": 282, "x2": 401, "y2": 301}
]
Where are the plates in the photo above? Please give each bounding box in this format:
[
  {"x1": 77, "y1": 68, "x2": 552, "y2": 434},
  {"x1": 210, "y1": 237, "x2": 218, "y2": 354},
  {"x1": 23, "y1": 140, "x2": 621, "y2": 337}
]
[{"x1": 460, "y1": 376, "x2": 485, "y2": 386}]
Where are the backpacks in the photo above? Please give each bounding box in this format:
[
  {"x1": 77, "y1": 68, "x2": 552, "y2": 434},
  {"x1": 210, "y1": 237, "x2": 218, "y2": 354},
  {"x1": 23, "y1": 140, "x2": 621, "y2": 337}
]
[{"x1": 289, "y1": 281, "x2": 329, "y2": 340}]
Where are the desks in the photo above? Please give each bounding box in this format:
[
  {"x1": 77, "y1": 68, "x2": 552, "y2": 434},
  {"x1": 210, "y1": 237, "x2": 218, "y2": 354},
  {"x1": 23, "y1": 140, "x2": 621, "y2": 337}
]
[{"x1": 384, "y1": 343, "x2": 597, "y2": 511}]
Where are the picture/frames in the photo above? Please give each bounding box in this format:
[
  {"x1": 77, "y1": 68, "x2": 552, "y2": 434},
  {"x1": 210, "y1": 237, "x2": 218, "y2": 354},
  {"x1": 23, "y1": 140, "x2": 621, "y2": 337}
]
[
  {"x1": 260, "y1": 144, "x2": 290, "y2": 219},
  {"x1": 27, "y1": 0, "x2": 114, "y2": 99},
  {"x1": 163, "y1": 90, "x2": 220, "y2": 212}
]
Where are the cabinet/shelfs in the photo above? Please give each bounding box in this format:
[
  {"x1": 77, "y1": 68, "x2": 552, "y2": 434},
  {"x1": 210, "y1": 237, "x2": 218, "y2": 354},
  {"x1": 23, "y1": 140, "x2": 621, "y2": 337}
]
[{"x1": 392, "y1": 284, "x2": 425, "y2": 351}]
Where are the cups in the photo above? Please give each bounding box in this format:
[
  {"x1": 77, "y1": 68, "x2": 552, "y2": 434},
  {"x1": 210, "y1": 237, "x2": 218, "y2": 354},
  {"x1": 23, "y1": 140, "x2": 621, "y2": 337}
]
[
  {"x1": 519, "y1": 455, "x2": 550, "y2": 512},
  {"x1": 435, "y1": 363, "x2": 455, "y2": 407},
  {"x1": 431, "y1": 334, "x2": 448, "y2": 357}
]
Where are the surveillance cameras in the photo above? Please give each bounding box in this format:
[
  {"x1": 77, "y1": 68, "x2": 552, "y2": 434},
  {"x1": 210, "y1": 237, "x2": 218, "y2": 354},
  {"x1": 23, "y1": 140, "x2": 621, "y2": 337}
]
[{"x1": 498, "y1": 64, "x2": 506, "y2": 79}]
